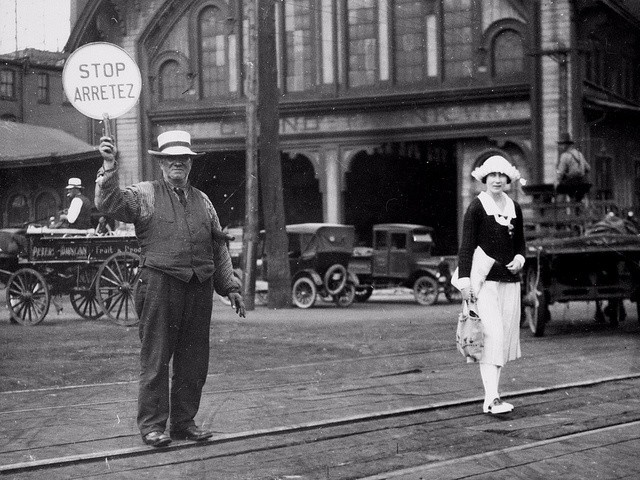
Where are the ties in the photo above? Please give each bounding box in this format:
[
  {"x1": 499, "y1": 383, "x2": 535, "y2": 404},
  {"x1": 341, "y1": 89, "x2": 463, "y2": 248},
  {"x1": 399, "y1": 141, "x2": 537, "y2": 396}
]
[{"x1": 174, "y1": 187, "x2": 187, "y2": 209}]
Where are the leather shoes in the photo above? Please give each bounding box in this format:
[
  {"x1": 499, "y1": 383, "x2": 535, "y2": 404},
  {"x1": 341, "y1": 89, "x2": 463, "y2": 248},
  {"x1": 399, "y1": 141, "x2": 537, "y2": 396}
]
[
  {"x1": 171, "y1": 425, "x2": 213, "y2": 441},
  {"x1": 143, "y1": 431, "x2": 172, "y2": 447}
]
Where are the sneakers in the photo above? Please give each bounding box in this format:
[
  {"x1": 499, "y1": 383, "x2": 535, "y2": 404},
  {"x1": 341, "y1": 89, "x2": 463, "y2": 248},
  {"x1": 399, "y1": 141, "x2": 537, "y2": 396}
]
[{"x1": 483, "y1": 393, "x2": 514, "y2": 414}]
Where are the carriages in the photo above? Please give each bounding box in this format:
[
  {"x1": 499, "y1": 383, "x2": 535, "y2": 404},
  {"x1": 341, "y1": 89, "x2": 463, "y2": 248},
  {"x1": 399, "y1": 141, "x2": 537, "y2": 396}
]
[
  {"x1": 513, "y1": 187, "x2": 640, "y2": 337},
  {"x1": 0, "y1": 209, "x2": 149, "y2": 326}
]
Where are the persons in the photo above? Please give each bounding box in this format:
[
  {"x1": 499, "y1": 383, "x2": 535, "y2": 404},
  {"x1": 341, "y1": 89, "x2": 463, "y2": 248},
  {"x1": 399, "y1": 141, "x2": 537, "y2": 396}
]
[
  {"x1": 457, "y1": 155, "x2": 527, "y2": 415},
  {"x1": 60, "y1": 177, "x2": 91, "y2": 230},
  {"x1": 94, "y1": 129, "x2": 247, "y2": 445},
  {"x1": 553, "y1": 132, "x2": 592, "y2": 217},
  {"x1": 95, "y1": 216, "x2": 112, "y2": 237}
]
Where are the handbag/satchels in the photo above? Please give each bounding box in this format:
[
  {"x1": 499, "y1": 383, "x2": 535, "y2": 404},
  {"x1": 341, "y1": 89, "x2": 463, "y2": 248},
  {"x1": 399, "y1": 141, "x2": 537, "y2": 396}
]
[{"x1": 456, "y1": 296, "x2": 485, "y2": 364}]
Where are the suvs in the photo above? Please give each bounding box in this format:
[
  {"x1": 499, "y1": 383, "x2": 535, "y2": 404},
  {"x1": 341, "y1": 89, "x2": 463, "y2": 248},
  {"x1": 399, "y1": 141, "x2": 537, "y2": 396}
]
[{"x1": 222, "y1": 223, "x2": 360, "y2": 307}]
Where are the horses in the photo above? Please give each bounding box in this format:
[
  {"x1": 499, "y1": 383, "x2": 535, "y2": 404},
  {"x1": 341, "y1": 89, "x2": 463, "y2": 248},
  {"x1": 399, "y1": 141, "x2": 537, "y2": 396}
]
[
  {"x1": 1, "y1": 231, "x2": 29, "y2": 323},
  {"x1": 594, "y1": 203, "x2": 639, "y2": 327}
]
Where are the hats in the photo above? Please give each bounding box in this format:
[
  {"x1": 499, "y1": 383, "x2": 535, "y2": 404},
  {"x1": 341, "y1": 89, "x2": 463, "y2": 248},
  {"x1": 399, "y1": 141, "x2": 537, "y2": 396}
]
[
  {"x1": 65, "y1": 177, "x2": 85, "y2": 189},
  {"x1": 148, "y1": 131, "x2": 206, "y2": 158},
  {"x1": 471, "y1": 155, "x2": 520, "y2": 184},
  {"x1": 556, "y1": 133, "x2": 574, "y2": 145}
]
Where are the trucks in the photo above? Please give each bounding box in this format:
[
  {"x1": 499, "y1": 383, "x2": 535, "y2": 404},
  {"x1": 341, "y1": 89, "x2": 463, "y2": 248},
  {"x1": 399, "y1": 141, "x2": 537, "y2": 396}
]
[{"x1": 349, "y1": 223, "x2": 462, "y2": 306}]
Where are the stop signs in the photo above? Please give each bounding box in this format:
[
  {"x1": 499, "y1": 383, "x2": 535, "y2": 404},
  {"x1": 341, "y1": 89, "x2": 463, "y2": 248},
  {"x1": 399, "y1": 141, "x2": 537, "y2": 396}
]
[{"x1": 61, "y1": 41, "x2": 144, "y2": 122}]
